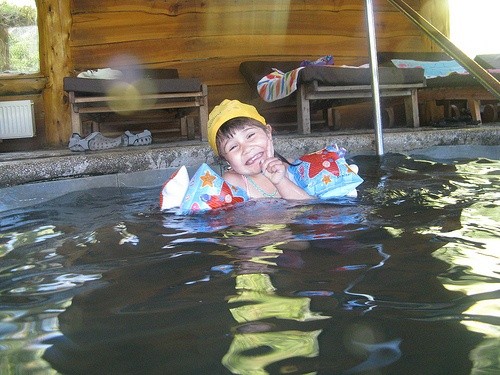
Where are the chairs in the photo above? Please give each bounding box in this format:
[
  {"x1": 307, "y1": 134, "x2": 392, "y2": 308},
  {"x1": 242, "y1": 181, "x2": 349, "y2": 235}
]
[
  {"x1": 62, "y1": 68, "x2": 209, "y2": 142},
  {"x1": 238, "y1": 51, "x2": 500, "y2": 135}
]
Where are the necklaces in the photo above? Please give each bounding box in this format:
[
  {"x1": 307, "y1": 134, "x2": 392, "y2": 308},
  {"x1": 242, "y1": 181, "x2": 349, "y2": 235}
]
[{"x1": 245, "y1": 175, "x2": 278, "y2": 198}]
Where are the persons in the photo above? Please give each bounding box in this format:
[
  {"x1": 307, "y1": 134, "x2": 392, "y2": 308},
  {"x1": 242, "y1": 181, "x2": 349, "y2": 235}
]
[{"x1": 207, "y1": 99, "x2": 316, "y2": 200}]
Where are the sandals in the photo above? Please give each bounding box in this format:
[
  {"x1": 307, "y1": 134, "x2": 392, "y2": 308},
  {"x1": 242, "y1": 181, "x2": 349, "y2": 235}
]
[
  {"x1": 115, "y1": 129, "x2": 152, "y2": 146},
  {"x1": 69, "y1": 131, "x2": 122, "y2": 151}
]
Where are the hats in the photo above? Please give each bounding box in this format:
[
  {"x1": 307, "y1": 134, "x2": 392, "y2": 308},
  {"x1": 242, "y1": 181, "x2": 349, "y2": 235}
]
[{"x1": 207, "y1": 98, "x2": 266, "y2": 157}]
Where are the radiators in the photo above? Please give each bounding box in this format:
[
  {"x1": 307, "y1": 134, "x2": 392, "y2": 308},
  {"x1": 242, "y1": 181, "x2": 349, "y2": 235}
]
[{"x1": 0, "y1": 100, "x2": 36, "y2": 140}]
